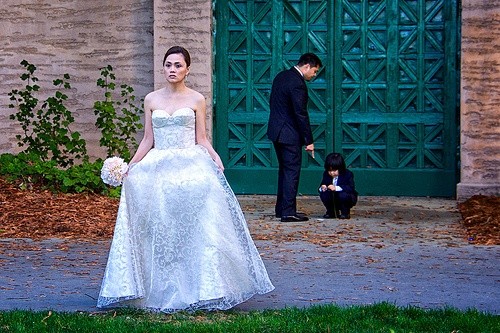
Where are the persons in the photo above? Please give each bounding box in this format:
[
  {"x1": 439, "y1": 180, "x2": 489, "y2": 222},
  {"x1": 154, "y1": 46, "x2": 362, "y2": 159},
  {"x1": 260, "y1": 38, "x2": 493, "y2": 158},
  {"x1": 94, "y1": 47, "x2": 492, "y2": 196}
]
[
  {"x1": 265, "y1": 51, "x2": 324, "y2": 222},
  {"x1": 96, "y1": 45, "x2": 276, "y2": 316},
  {"x1": 317, "y1": 151, "x2": 360, "y2": 218}
]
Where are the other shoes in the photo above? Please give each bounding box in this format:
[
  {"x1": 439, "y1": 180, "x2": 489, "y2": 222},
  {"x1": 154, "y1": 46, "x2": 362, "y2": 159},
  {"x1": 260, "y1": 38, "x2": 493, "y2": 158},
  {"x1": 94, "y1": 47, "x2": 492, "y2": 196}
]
[
  {"x1": 281, "y1": 214, "x2": 309, "y2": 222},
  {"x1": 275, "y1": 212, "x2": 307, "y2": 218},
  {"x1": 339, "y1": 214, "x2": 351, "y2": 219},
  {"x1": 323, "y1": 212, "x2": 335, "y2": 218}
]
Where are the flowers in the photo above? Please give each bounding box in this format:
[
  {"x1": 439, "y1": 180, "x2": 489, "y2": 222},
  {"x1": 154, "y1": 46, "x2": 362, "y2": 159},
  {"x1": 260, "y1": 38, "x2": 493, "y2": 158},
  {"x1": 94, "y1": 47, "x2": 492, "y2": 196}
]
[{"x1": 100, "y1": 156, "x2": 129, "y2": 186}]
